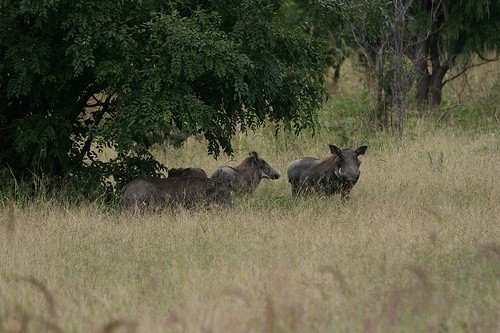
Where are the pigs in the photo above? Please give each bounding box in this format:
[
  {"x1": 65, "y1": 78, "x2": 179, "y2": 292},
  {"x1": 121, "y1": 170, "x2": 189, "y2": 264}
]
[
  {"x1": 286, "y1": 143, "x2": 369, "y2": 206},
  {"x1": 121, "y1": 152, "x2": 280, "y2": 213}
]
